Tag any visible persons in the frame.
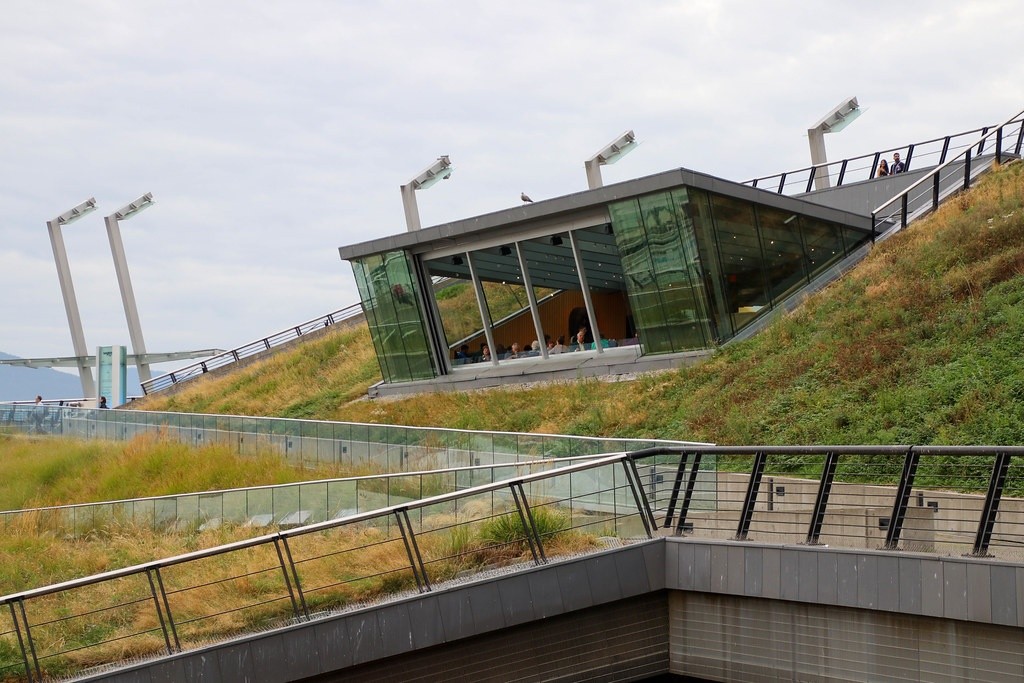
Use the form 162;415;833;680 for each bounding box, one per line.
877;159;889;177
97;396;109;409
889;153;906;175
33;396;46;434
454;328;621;363
